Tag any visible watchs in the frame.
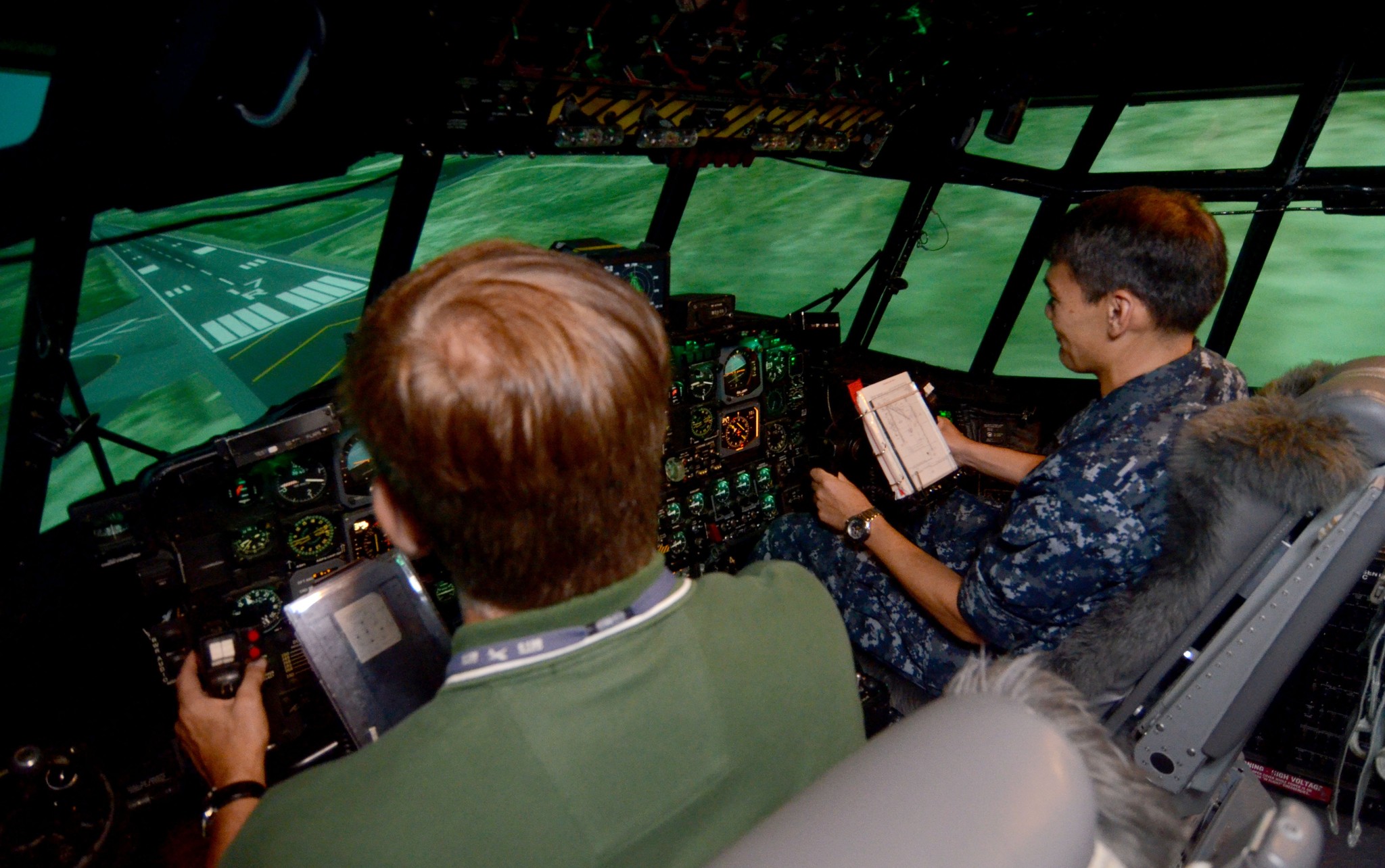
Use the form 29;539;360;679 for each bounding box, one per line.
200;780;266;838
843;508;881;550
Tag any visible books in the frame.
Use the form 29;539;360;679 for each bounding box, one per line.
856;370;958;500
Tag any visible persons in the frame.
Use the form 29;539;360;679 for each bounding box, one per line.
178;239;868;868
812;191;1250;697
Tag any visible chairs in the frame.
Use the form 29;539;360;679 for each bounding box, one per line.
1065;354;1384;820
706;649;1323;868
